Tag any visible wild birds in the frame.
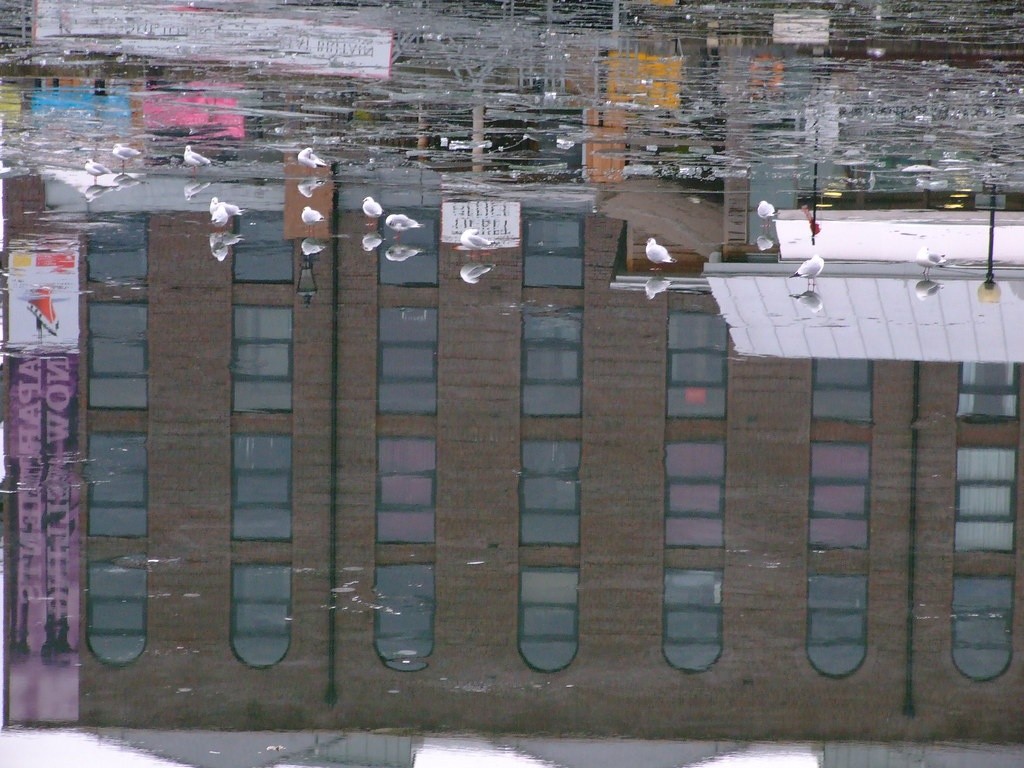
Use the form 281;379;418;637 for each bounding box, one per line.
645;238;677;269
84;170;148;202
301;206;329;232
300;233;329;257
207;197;245;226
915;246;945;276
183;145;211;170
756;228;773;251
83;143;143;181
362;225;426;263
788;285;825;317
209;231;246;262
644;276;672;300
459;255;497;284
460;227;495;249
786;253;825;285
757;199;779;227
183;177;211;200
914;276;944;302
297;146;328;173
297;173;329;198
362;196;425;239
